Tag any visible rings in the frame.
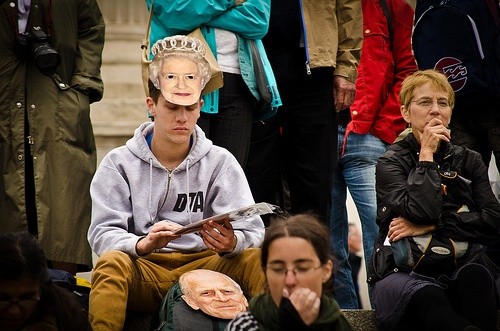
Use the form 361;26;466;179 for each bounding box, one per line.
211;247;216;251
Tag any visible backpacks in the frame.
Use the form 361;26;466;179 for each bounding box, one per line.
393;167;484;281
158;279;228;331
411;0;491;98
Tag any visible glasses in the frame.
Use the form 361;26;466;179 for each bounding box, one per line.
410;99;450;107
0;297;37;310
263;265;323;277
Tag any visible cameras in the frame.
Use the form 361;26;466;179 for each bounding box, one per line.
14;26;62;77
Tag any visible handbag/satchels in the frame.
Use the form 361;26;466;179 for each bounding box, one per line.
139;2;223;98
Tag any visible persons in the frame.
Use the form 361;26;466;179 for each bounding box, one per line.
146;1;499;308
367;71;500;331
0;0;105;267
0;231;94;331
87;36;264;331
225;214;355;331
